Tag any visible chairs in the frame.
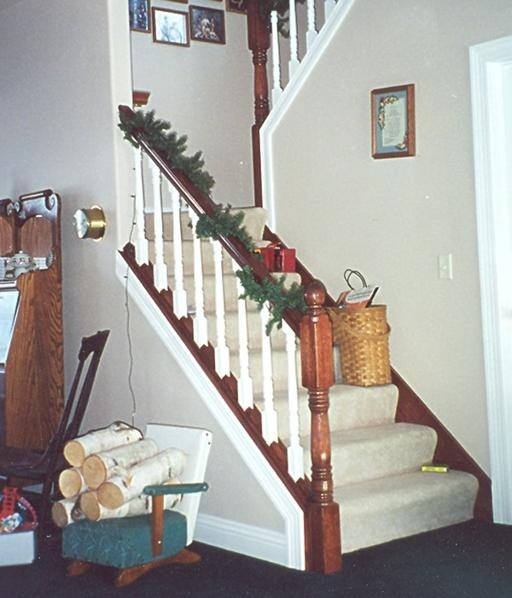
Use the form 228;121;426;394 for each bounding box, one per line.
1;331;107;560
62;425;215;586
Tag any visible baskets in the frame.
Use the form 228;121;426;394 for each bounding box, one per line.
327;306;392;386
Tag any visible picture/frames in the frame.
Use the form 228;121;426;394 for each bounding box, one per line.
129;0;248;48
371;83;416;159
